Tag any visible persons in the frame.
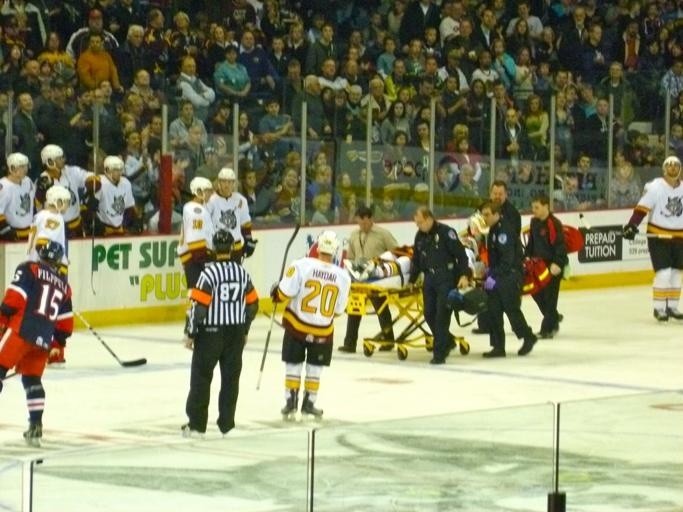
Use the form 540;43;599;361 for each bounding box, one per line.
27;186;71;368
305;180;569;364
0;240;74;438
181;229;258;439
176;168;258;299
270;232;351;420
622;156;683;322
1;2;682;242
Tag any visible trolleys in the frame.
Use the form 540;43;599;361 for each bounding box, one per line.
352;274;474;360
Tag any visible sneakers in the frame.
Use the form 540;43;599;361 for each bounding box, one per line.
343;258;379;283
180;422;205;433
22;420;43;440
653;307;683;321
336;344;356;354
377;342;394;352
429;313;566;364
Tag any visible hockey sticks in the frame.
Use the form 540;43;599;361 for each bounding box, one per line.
255;223;301;391
73;309;146;368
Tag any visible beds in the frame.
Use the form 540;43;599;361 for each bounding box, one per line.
347;246;546;360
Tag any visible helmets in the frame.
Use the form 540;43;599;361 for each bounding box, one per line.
661;155;681;170
39;144;64;166
5;152;28;167
44;184;71;205
316;231;339;255
38;241;63;261
216;166;236;180
189;176;212;194
103;155;124;171
212;231;233;248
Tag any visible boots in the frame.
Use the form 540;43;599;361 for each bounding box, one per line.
279;386;324;417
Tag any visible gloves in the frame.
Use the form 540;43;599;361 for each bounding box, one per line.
46;340;64;364
483;277;496;291
81;192;98;212
241;238;258;257
622;224;638;241
34;176;53;204
